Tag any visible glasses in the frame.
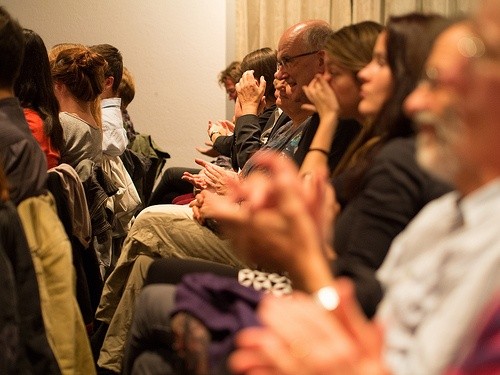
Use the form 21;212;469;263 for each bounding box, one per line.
278;51;318;70
